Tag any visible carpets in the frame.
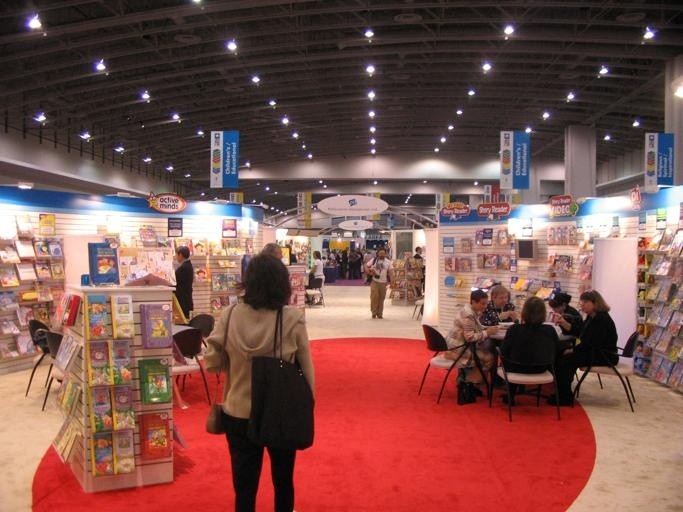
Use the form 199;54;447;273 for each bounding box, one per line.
32;337;595;512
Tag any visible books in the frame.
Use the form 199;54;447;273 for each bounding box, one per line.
54;294;82;462
632;201;683;395
85;295;171;476
87;243;120;285
104;224;170;279
0;213;64;359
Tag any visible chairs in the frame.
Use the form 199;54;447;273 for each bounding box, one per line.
418;324;492;403
571;330;639;413
307;278;325;309
487;337;565;422
42;331;62;411
412;296;424;320
171;292;221;410
25;319;53;397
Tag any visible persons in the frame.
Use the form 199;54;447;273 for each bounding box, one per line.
439;289;504;386
545;288;618;409
546;290;582;335
479;284;519;350
306;244;377;290
173;245;193;319
497;294;561;407
261;242;282;261
202;252;317;512
411;245;425;297
362;248;396;319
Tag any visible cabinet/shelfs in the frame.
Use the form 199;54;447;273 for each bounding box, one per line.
188;256;244;326
52;283;177;494
389;248;424;306
633;219;683;395
286;266;306;309
0;234;67;375
59;235;179;289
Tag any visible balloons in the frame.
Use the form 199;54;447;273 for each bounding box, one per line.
442;221;623;302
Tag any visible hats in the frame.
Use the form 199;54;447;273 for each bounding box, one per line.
549;292;571;308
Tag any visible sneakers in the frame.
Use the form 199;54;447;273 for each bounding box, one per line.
371;313;383;319
457;367;573;407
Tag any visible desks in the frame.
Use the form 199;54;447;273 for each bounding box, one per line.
306;288;321;295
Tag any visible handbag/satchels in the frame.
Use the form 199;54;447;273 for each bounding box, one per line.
248;304;315;453
206;402;229;433
366;271;372;282
